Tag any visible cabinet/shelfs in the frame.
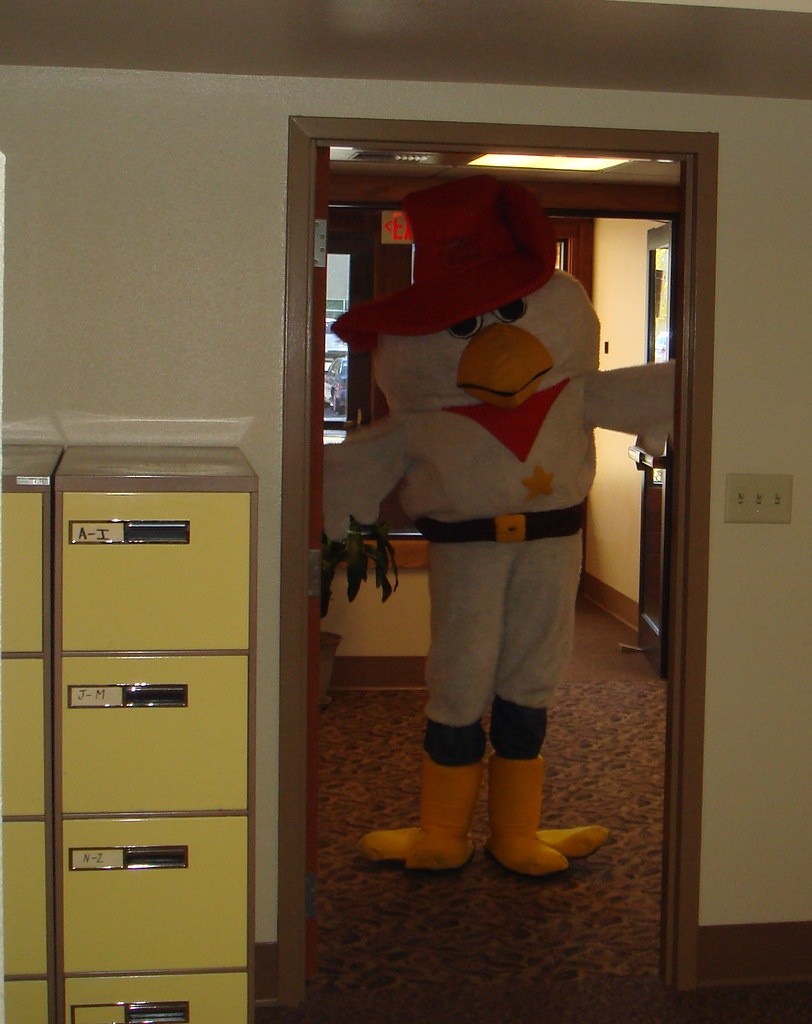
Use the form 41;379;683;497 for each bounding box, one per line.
0;443;65;1024
53;445;260;1024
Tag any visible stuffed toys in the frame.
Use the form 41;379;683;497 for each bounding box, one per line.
323;174;676;876
